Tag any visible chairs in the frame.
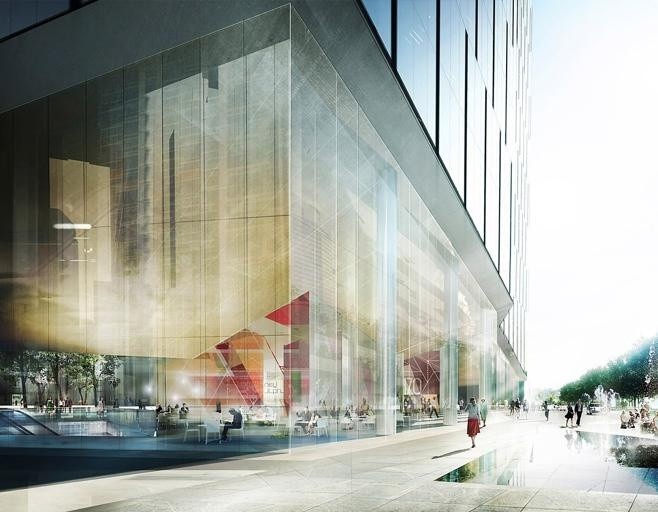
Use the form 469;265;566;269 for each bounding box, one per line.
156;406;376;445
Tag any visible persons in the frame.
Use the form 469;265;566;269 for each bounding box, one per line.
563;402;573;427
573;398;583;426
617;400;657;435
513;398;521;420
508;400;514;416
458;398;464;414
479;398;488;428
541;400;549;421
521;399;529;420
459;396;480;448
426;398;431;413
428;396;439;419
403;398;408;416
406;396;413;416
